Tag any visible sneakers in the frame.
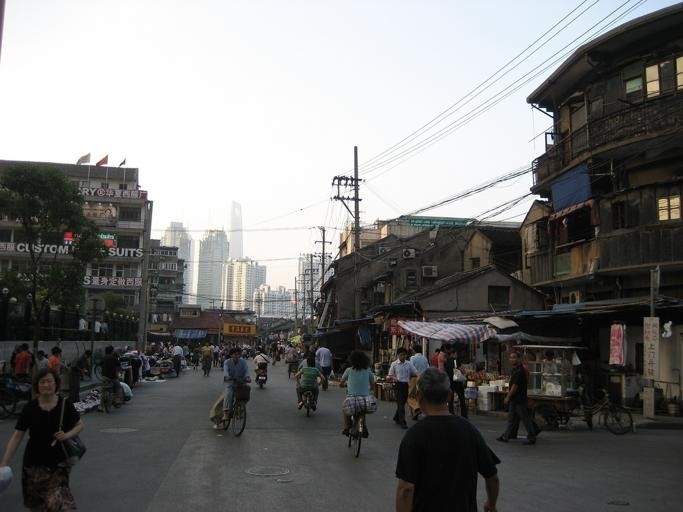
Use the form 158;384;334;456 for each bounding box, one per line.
221;416;229;421
298;402;304;410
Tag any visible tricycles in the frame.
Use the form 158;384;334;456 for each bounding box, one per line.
0;358;32;420
489;375;634;436
288;359;301;380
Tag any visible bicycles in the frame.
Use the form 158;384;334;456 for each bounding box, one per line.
217;374;254;438
92;352;104;381
339;380;373;459
295;375;320;418
99;366;127;414
172;342;264;377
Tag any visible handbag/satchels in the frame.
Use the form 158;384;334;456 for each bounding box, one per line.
453;368;466;382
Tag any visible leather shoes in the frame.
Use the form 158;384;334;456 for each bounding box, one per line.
496;436;507;442
342;430;349;436
401;422;408;429
393;417;399;424
523;437;537;445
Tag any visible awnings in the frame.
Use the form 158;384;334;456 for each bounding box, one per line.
175;328;207;338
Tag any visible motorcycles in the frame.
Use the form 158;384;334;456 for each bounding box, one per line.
252;361;268;388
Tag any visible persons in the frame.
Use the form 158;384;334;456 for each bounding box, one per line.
241;339;286;384
394;366;501;512
76;346;126;412
1;367;84;512
223;348;251;421
495;350;559;445
284;343;333;412
340;350;375;438
145;341;240;377
387;343;468;429
9;341;64;378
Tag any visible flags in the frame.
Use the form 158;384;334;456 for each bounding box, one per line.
76;152;90;166
118;159;124;167
95;155;107;166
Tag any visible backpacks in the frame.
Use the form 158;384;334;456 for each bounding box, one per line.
50;397;86;464
